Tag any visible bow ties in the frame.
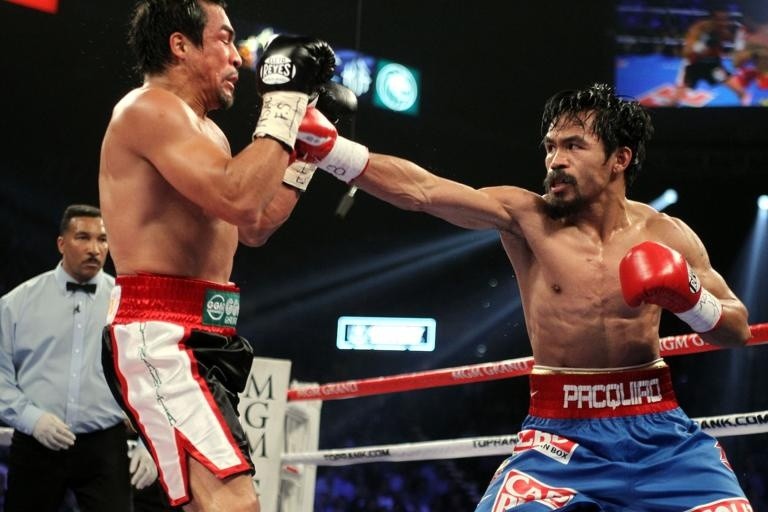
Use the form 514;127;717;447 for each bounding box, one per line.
66;282;96;293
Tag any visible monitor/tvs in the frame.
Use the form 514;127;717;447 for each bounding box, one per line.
605;0;768;113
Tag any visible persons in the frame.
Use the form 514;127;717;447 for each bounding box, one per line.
0;205;157;512
726;5;768;105
671;7;752;107
302;82;753;510
100;0;359;512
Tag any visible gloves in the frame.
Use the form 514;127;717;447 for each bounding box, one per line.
287;107;369;183
282;81;357;193
129;438;158;489
619;242;724;334
252;30;336;150
33;414;76;450
